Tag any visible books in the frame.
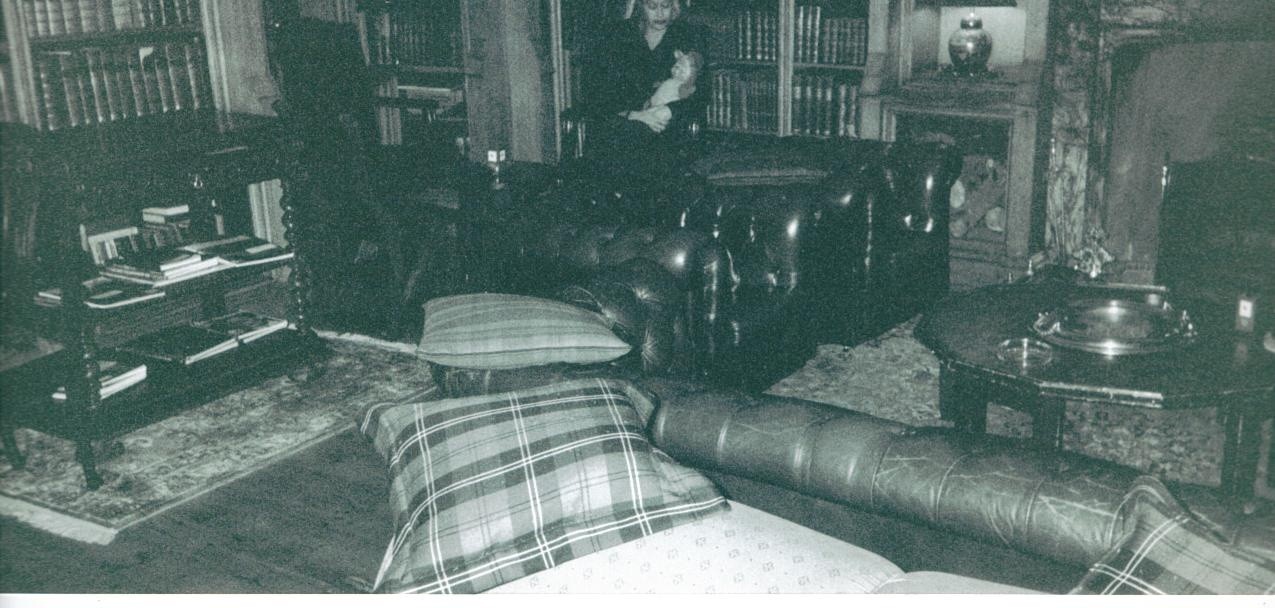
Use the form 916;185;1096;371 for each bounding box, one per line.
0;0;462;403
696;4;867;138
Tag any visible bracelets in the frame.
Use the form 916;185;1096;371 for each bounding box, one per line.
627;108;633;119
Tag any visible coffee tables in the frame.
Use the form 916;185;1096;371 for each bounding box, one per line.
912;274;1275;496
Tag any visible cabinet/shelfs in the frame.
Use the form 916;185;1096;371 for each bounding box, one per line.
557;0;872;141
0;0;223;132
25;107;323;440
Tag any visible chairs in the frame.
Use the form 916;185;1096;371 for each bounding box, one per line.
0;117;99;494
1154;152;1275;298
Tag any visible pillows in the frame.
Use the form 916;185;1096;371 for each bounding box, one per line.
361;371;735;594
1066;470;1275;595
410;279;638;368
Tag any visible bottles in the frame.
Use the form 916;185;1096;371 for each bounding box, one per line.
948;15;993;76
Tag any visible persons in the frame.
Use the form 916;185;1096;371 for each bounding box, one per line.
589;1;708;170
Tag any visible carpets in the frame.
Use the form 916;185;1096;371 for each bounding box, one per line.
0;311;433;548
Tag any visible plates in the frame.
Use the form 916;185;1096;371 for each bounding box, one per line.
1033;299;1198;356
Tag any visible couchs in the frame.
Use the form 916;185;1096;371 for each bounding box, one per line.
314;115;1275;595
